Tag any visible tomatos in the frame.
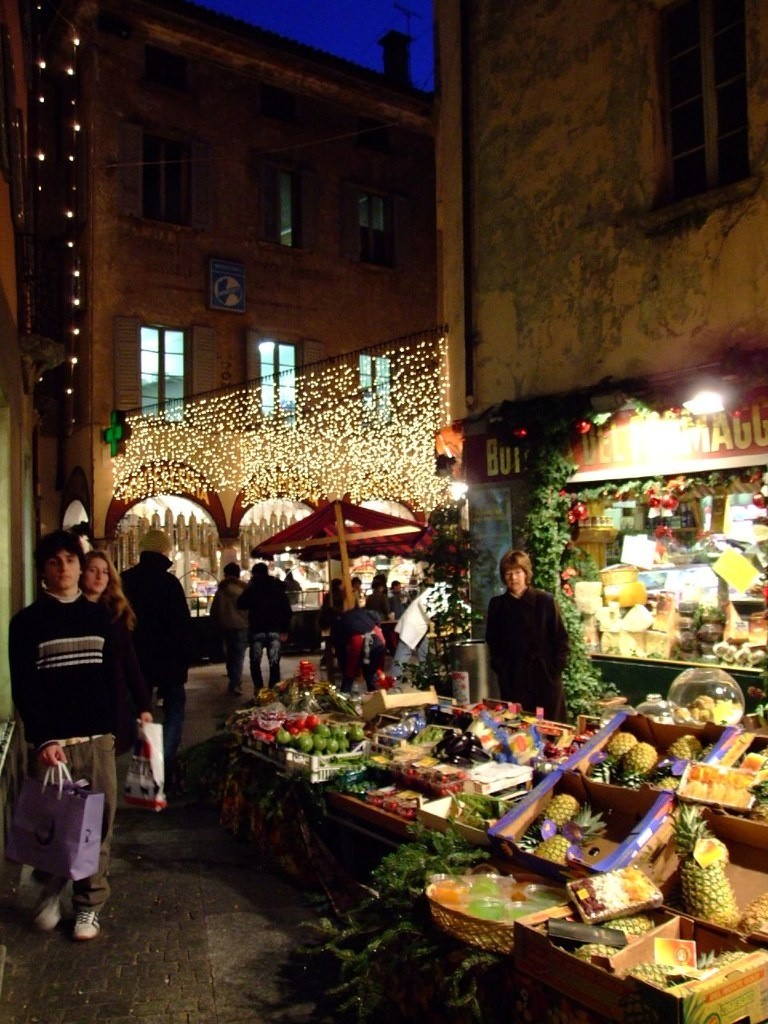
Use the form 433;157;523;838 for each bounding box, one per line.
366;766;468;819
250;714;320;743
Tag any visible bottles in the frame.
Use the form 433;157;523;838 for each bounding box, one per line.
668;668;744;726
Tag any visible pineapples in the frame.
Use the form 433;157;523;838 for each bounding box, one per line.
520;730;768;993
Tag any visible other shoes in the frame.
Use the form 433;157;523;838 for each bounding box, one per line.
230;686;244;696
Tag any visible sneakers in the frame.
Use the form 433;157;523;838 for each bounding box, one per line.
73;910;102;941
32;896;62;930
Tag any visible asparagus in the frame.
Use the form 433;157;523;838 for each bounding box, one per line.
284;683;358;717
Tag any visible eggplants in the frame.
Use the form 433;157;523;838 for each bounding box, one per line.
431;730;492;767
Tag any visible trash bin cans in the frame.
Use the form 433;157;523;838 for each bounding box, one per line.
455;639;490;704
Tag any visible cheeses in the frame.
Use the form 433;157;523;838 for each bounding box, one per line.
571;565;676;661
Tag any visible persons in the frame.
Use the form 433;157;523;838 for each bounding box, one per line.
284;568;302;604
237;563;292;697
120;529;193;801
78;550;154;786
210;563;249;695
485;550;571;723
318;574;417;693
9;530;119;941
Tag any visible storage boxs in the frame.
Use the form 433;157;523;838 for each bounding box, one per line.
362;684;768;1024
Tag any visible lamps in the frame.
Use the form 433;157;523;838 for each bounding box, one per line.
682;380;725;415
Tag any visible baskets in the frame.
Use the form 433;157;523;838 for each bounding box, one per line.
424;875;569;955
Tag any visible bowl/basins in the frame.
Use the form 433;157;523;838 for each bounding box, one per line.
428;871;570;923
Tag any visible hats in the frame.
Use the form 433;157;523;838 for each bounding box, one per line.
141;530;171;554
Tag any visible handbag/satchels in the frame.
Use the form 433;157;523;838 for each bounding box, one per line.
123;719;169;813
5;759;106;882
